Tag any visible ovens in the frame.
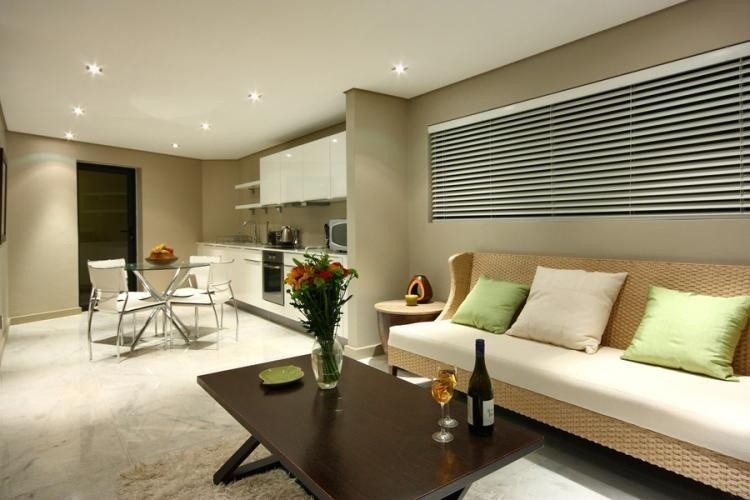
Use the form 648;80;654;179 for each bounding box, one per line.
262;250;284;307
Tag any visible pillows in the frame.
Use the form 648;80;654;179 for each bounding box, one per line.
450;275;530;334
505;265;628;353
621;284;749;382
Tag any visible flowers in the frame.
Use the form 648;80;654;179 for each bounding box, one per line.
284;249;360;384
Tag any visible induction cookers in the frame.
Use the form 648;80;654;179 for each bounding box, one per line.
264;246;305;249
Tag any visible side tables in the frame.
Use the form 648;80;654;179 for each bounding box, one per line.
374;298;446;355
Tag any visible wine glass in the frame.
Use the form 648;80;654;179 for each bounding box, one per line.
431;378;454;443
438;368;458;428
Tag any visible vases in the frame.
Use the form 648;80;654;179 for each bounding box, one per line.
311;336;343;390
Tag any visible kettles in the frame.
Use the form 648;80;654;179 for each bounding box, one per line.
280;225;294;246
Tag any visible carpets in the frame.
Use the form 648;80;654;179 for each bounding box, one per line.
113;430;506;499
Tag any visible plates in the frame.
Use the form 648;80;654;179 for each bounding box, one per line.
259;365;304;385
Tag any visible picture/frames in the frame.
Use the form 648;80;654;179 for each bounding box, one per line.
1;148;6;244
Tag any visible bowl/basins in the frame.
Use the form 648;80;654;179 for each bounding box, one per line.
144;257;178;266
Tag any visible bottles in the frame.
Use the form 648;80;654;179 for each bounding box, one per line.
466;338;494;435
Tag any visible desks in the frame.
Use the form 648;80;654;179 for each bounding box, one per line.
124;261;210;352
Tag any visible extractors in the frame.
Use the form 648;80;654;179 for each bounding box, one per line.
263;203;329;208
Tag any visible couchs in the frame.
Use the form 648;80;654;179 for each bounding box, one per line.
387;250;750;500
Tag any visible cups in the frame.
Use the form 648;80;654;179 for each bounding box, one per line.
405;294;419;306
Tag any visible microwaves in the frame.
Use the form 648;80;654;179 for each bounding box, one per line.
329;219;347;253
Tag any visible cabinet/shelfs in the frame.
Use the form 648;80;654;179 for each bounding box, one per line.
196;245;216;284
303;131;347;203
234;180;260;210
216;246;262;304
283;252;348;349
260;144;303;208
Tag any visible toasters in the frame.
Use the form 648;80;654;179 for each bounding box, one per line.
269;231;282;245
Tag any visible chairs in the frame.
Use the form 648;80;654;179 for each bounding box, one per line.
163;256;221;338
87;258;166;345
87;264;166;359
168;260;238;349
